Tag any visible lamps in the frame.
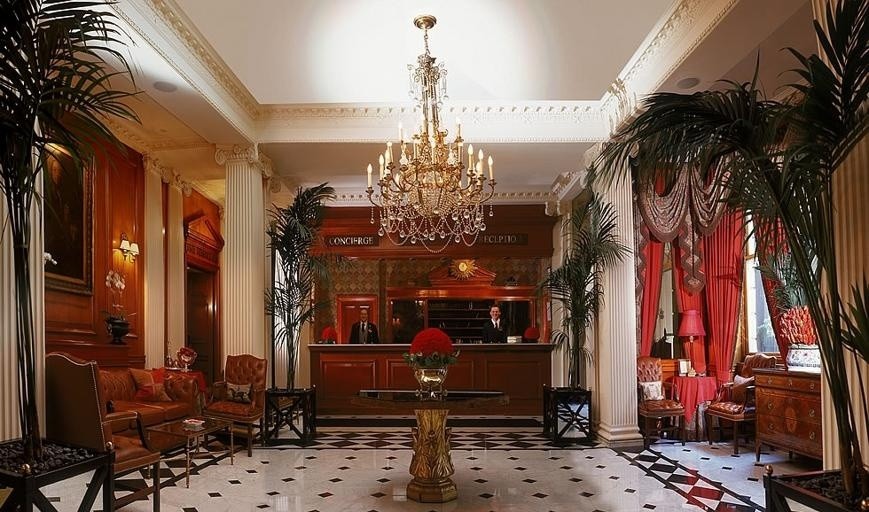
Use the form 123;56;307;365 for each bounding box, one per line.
363;12;498;254
119;232;139;263
677;309;707;378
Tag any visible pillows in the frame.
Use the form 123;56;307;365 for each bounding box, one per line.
128;368;172;403
224;380;254;405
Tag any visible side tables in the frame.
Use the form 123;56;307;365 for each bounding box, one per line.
671;374;718;446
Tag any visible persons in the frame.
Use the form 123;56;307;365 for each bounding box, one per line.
43;154;84;273
482;304;508;343
349;308;380;344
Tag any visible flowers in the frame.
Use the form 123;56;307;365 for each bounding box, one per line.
321;326;337;343
403;326;462;367
524;326;540;340
778;305;817;344
102;267;137;321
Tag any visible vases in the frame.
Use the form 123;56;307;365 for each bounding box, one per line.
104;317;129;345
413;368;449;398
784;344;821;374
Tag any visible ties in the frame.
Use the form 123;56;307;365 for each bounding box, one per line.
495;323;497;328
361;324;365;331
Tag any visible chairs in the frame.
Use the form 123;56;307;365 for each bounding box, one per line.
42;351;162;512
705;355;778;454
202;353;268;458
638;356;688;448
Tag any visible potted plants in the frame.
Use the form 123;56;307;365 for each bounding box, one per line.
528;181;634;444
262;180;336;448
0;0;146;512
583;2;869;512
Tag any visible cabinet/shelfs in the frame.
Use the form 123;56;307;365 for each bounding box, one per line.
754;368;822;466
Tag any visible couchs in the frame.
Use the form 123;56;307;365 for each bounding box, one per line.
96;363;208;440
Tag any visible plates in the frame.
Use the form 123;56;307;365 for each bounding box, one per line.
183;427;205;432
183;418;204;427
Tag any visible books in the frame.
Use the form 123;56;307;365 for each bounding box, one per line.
507;334;524;343
